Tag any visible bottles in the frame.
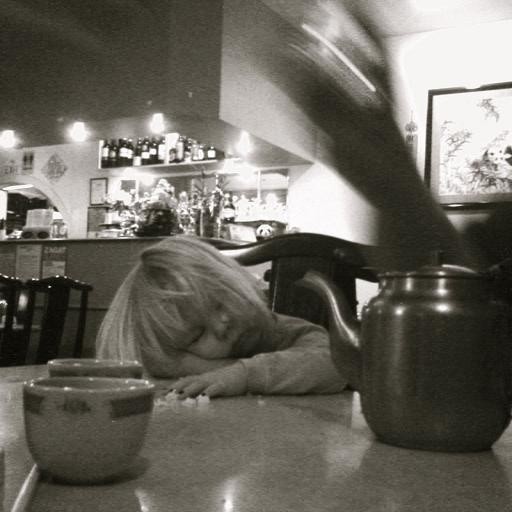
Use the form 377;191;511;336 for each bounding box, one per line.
222;194;234;221
199;207;212;237
100;135;218;169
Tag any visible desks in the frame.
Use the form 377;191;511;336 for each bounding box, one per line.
1;363;511;511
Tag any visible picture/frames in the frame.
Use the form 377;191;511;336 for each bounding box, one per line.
424;81;512;211
90;178;108;206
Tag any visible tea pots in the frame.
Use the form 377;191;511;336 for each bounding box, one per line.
294;252;512;452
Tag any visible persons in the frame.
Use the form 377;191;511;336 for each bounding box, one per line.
95;234;349;401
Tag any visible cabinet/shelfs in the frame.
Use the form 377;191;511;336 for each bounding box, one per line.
98;140;290;241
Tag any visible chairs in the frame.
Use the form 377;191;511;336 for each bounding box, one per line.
24;275;98;363
219;233;445;332
0;274;28;367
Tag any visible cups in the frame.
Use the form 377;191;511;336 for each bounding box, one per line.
21;376;156;485
47;358;144;379
